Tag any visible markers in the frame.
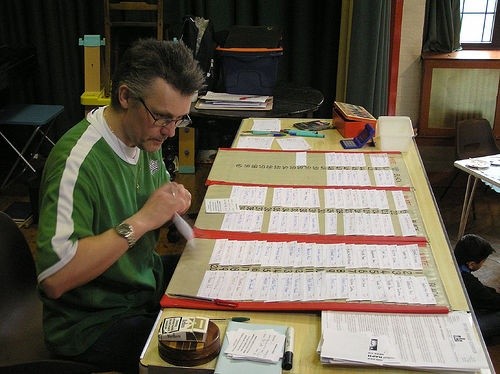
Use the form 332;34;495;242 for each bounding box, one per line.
282;326;294;370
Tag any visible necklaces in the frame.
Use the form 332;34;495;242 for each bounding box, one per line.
104;108;140;188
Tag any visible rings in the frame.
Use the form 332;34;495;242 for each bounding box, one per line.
171;192;175;196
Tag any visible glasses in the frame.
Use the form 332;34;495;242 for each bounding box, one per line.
138;97;192;127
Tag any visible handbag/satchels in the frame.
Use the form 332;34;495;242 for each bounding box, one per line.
177;15;216;95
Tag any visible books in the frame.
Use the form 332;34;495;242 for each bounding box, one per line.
199;91;269;107
214;321;288;374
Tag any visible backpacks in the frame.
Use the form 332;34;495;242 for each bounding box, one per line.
215;25;287;96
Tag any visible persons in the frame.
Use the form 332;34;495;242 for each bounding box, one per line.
454;234;500;337
34;40;209;374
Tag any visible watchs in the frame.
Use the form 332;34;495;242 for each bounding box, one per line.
115;223;136;248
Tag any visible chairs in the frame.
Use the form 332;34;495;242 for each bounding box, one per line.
438;119;500;221
0;211;107;374
217;24;284;91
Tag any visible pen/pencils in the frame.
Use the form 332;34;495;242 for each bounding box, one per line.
209;316;250;322
253;129;325;138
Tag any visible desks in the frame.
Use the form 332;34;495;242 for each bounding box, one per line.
138;115;496;374
454;154;500;241
189;87;325;150
0;103;65;191
